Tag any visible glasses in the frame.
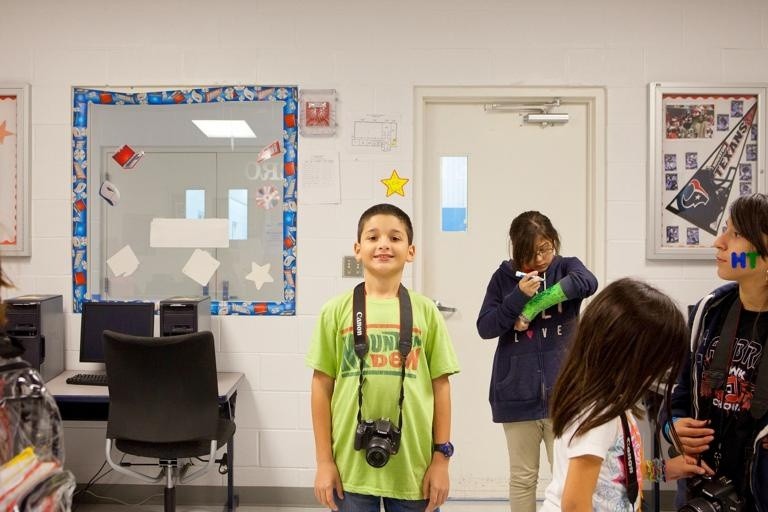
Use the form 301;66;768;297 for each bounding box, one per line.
532;239;554;256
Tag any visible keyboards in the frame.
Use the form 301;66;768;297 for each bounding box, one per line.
66;374;109;385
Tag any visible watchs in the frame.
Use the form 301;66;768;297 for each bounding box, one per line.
431;439;456;459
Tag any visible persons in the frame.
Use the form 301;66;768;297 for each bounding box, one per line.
662;100;758;247
303;203;461;512
535;276;717;510
475;209;600;511
656;190;767;510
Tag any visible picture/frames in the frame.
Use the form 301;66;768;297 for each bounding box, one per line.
0;81;34;260
644;79;768;263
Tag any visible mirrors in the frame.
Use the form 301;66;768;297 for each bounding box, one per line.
71;83;300;317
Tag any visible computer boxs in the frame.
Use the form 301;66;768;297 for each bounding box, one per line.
3;294;64;385
160;296;210;337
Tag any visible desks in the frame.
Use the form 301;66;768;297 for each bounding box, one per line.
45;370;243;510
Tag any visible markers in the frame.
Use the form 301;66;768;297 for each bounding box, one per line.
515;271;546;282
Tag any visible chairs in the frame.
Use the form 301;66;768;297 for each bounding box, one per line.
93;328;236;511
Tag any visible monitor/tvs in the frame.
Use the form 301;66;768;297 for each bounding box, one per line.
79;302;154;363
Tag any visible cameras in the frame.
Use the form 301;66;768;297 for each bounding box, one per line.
677;472;743;512
353;419;401;468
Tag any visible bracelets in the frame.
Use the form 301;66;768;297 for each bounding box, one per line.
663;416;681;443
644;457;668;483
